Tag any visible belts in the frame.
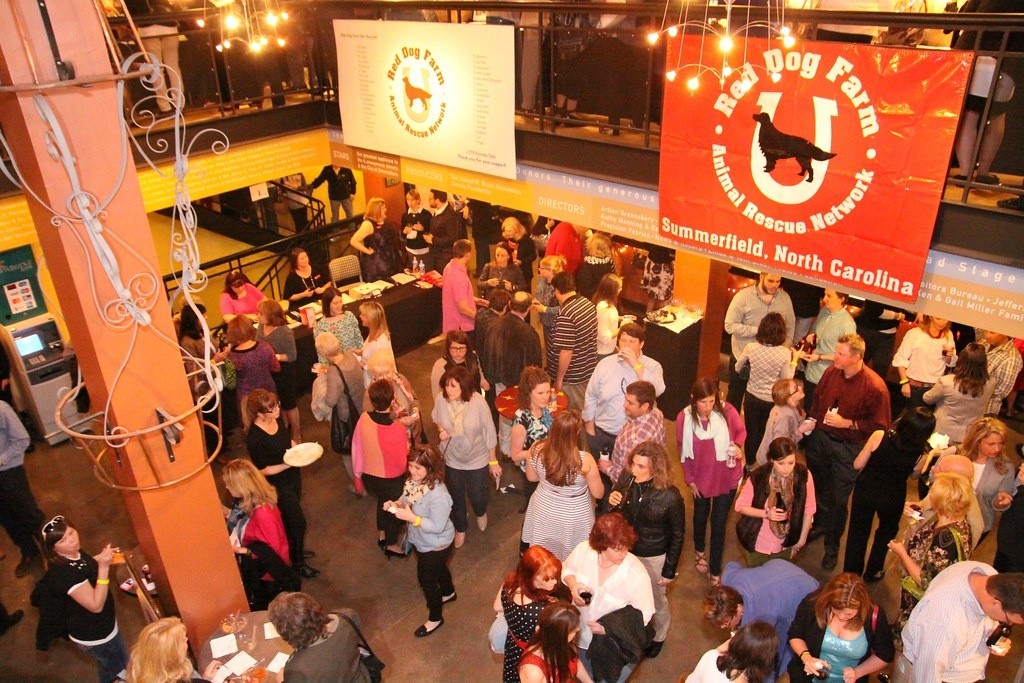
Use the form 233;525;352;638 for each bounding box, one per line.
908;377;935;388
823;430;852;444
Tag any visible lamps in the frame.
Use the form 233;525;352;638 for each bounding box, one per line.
646;0;795;94
195;0;289;53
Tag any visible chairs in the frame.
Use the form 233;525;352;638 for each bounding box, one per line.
329;254;366;291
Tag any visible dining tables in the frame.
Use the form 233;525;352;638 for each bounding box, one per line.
643;306;701;421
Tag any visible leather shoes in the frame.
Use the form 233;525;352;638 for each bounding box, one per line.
15;555;35;578
218;442;243;465
302;552;320;579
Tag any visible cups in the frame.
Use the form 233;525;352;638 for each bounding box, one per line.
803;420;812;436
814;661;831;680
580;592;592;605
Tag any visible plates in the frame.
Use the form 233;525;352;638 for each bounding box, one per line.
283;442;324;467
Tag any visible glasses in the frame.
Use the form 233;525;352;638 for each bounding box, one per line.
449;346;467;352
790;382;798;396
265;400;281;413
42;515;64;541
995;597;1015;628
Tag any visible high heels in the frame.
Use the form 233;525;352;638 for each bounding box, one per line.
377;539;411;561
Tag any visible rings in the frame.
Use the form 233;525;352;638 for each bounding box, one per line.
442;436;445;439
498;469;501;472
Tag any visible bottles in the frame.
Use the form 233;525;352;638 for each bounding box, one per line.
806;334;817;354
823;398;840;425
793;339;804;350
419;260;425;275
412;257;418;273
409;397;418;416
727;441;736;468
607;476;635;513
219;333;226;353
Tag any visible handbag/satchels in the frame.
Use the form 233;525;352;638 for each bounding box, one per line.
899;526;964;601
330;363;360;455
328;612;385;683
740;360;751;382
224;359;236;389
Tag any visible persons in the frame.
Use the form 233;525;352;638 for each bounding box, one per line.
675;271;1024;683
116;617;222;683
950;0;1024;188
308;165;357;223
62;339;91;420
181;190;686;683
552;0;639;127
0;400;45;578
281;173;311;232
0;602;24;635
30;515;128;683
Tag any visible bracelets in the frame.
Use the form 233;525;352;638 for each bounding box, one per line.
899;380;909;384
849;420;855;430
800;650;809;658
766;508;769;517
439;427;445;432
97;579;110;585
321;288;326;291
634;365;642;369
489;462;498;464
790;362;797;365
413;517;420;526
818;354;821;362
275;353;281;361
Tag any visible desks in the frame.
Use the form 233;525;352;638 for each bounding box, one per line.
218;269;442;398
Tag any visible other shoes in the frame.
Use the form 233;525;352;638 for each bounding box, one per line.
476;513;488;531
414;618;444;637
442;592;457;605
863;569;885;582
0;609;24;636
347;484;367;498
806;525;826;545
645;640;664;658
822;553;838;571
454;530;465;548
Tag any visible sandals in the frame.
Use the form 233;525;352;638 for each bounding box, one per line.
710;574;720;587
694;550;708;574
